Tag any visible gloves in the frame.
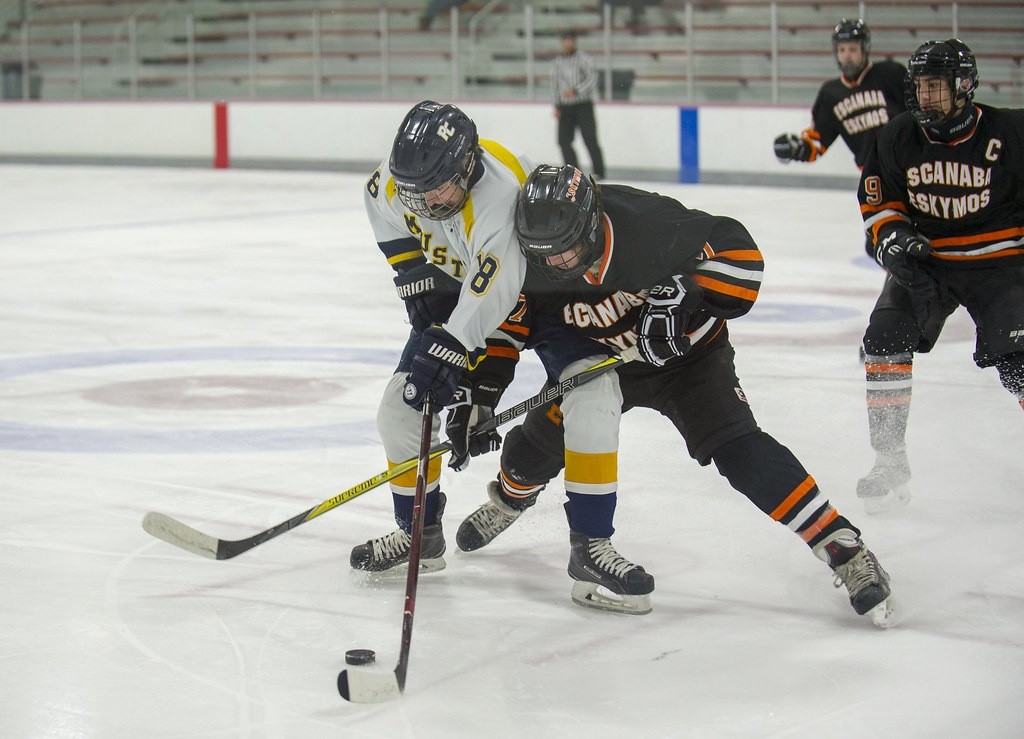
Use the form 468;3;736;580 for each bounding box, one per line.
394;265;458;336
775;134;814;162
445;379;505;471
872;231;935;298
636;269;705;367
403;324;472;415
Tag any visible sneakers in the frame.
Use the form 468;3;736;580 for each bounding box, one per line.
350;491;446;582
811;536;894;629
563;501;656;616
856;453;910;516
454;472;527;555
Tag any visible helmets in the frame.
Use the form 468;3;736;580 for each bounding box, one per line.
901;37;978;130
390;99;480;222
832;17;873;81
515;164;599;283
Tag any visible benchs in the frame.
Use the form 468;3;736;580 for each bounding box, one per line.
0;0;1024;108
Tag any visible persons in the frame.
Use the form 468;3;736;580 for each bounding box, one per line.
550;30;607;179
351;97;656;615
774;17;917;257
855;40;1024;499
456;163;894;629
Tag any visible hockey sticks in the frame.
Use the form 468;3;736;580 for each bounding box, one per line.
334;390;436;703
140;343;644;563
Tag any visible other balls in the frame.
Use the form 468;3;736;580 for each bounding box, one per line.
345;649;376;666
